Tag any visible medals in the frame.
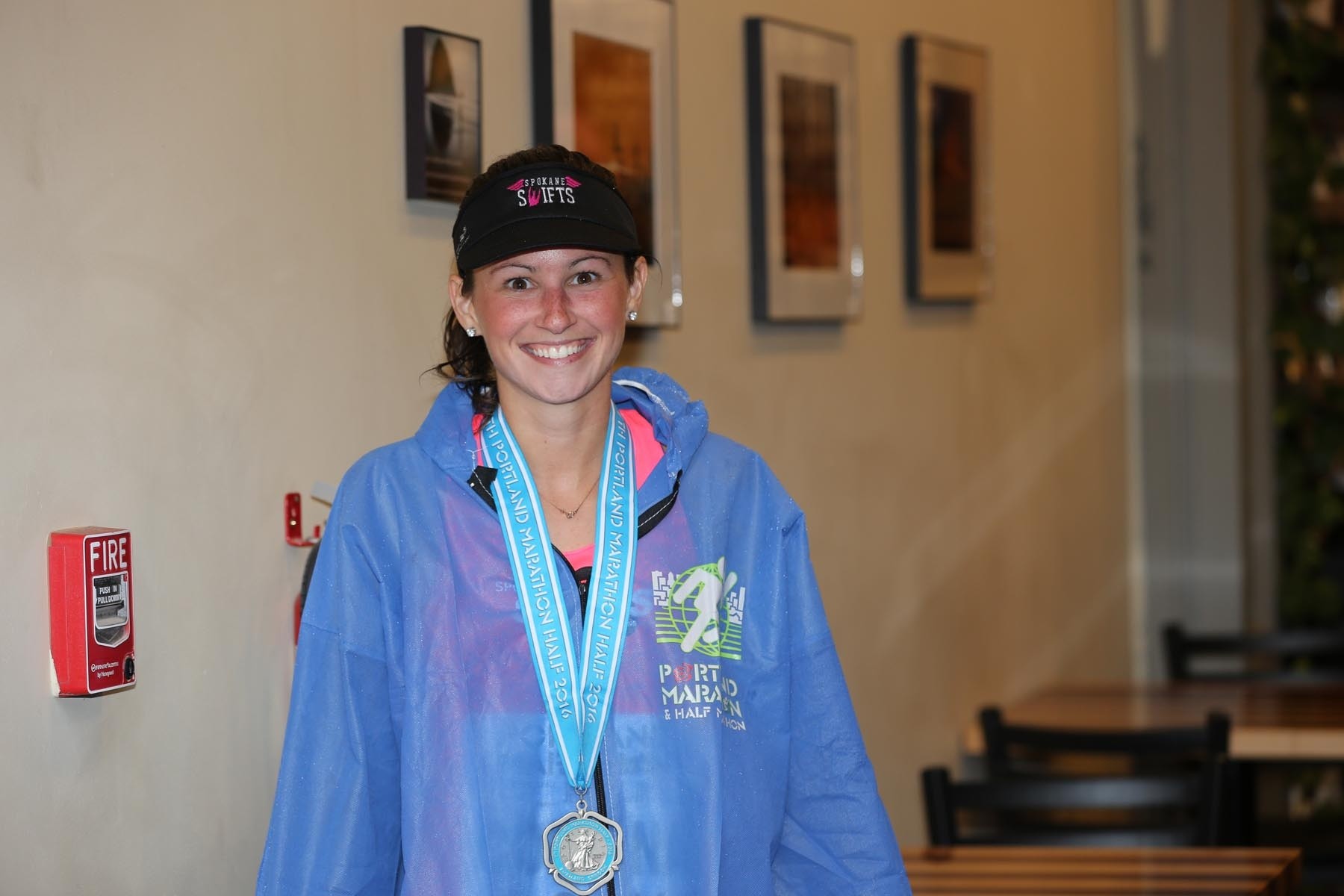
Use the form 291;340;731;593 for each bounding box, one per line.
543;801;623;896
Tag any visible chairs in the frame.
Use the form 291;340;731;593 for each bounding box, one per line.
913;587;1344;857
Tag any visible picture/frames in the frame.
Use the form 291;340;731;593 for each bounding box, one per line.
735;11;875;334
400;24;486;215
893;29;996;311
531;0;685;342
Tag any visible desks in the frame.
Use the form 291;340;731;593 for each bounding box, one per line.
943;668;1344;764
897;830;1307;896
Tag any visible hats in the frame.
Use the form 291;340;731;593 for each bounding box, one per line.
452;162;637;277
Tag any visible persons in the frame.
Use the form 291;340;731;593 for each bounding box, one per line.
255;147;913;896
